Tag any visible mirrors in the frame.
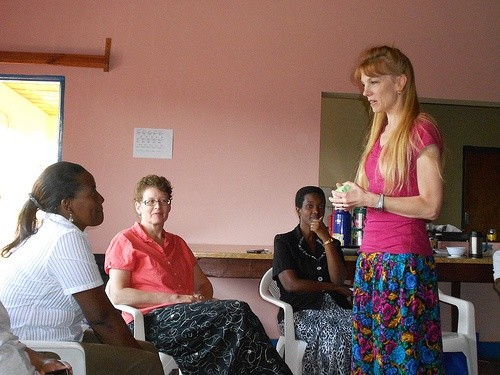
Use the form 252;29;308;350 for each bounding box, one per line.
314;86;500;243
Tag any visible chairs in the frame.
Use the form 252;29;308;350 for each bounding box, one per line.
260;259;481;375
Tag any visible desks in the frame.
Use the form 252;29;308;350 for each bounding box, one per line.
193;249;500;347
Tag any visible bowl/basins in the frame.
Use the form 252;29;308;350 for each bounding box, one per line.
446;246;466;256
433;249;448;254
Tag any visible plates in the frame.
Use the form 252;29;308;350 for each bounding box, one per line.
448;256;464;258
434;254;448;257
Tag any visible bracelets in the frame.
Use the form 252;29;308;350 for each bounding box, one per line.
321;238;334;246
375;193;384;211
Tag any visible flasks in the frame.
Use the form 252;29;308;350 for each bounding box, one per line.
469;231;482;258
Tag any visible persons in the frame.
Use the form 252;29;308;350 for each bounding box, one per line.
1;302;73;375
104;174;298;375
328;45;446;375
0;161;165;375
273;186;354;375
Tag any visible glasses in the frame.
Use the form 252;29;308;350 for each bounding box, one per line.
140;198;173;206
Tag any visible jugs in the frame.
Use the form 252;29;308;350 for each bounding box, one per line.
487;228;496;243
349;205;365;246
328;209;336;238
334;207;350;245
